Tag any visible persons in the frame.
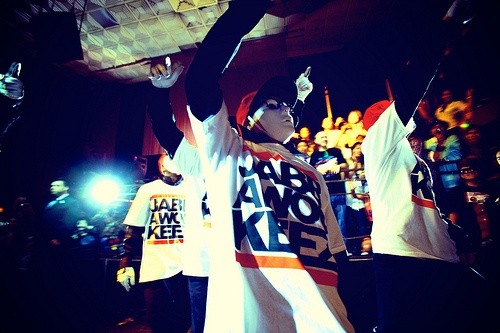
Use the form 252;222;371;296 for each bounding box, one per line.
118;149;182;332
184;0;357;333
360;0;474;333
0;60;25;135
147;56;313;332
289;89;498;257
39;180;75;331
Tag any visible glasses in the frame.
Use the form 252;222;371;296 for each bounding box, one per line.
251;97;293;114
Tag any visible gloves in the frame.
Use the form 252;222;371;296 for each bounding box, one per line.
148;56;184;88
295;66;313;103
117;267;135;292
0;63;24;99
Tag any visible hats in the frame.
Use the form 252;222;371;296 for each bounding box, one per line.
362;100;392;131
236;76;298;127
